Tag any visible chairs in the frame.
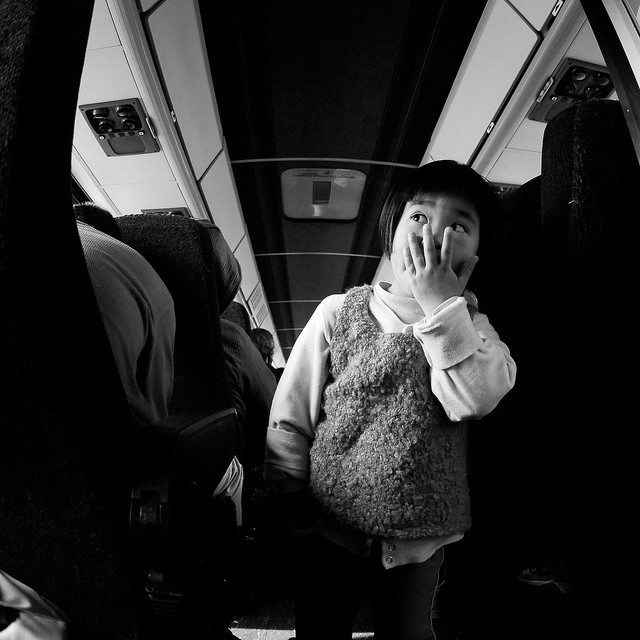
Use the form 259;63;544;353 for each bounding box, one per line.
542;101;639;324
0;2;241;639
116;217;236;410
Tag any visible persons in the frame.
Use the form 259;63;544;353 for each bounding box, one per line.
215;319;277;468
261;160;519;638
248;329;285;385
70;202;244;639
